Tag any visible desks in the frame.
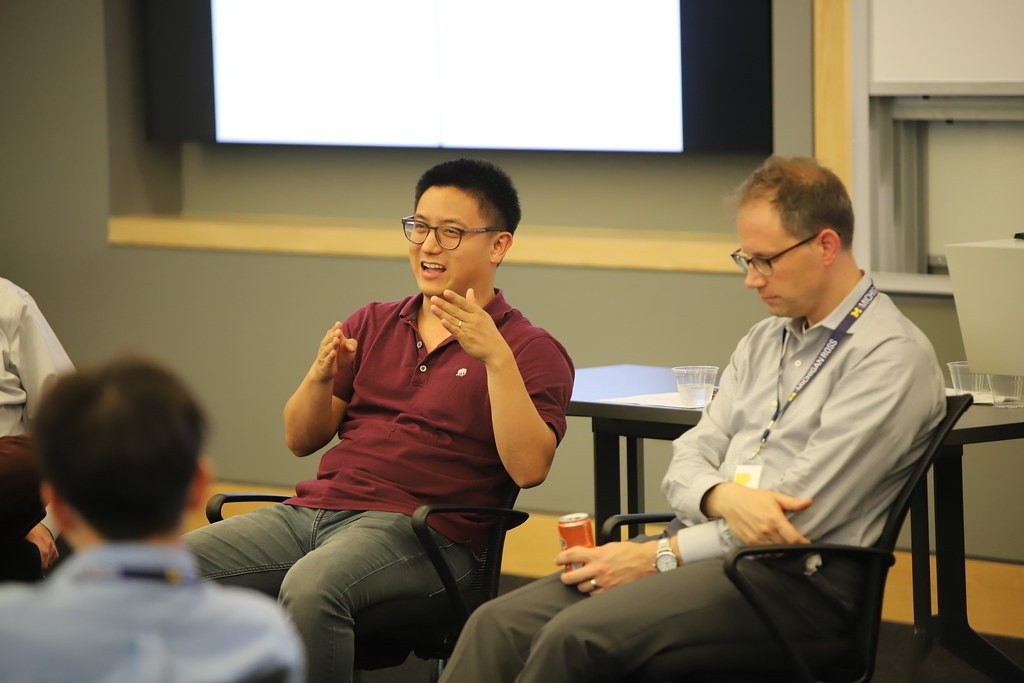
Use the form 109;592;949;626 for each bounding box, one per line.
565;363;1024;682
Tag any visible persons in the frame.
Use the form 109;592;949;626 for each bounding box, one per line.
1;360;306;683
178;158;574;683
437;154;946;683
1;277;81;571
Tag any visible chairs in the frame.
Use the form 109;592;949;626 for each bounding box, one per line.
204;493;532;683
602;392;974;683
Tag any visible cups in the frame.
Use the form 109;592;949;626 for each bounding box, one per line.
672;366;719;410
987;374;1024;408
947;360;983;396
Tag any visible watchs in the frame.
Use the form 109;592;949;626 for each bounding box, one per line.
652;537;678;573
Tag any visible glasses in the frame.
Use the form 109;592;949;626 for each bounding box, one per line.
400;216;504;250
731;232;841;277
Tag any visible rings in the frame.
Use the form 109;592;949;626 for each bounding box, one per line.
459;321;462;328
590;579;598;589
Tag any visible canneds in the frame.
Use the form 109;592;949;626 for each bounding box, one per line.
558;512;596;577
